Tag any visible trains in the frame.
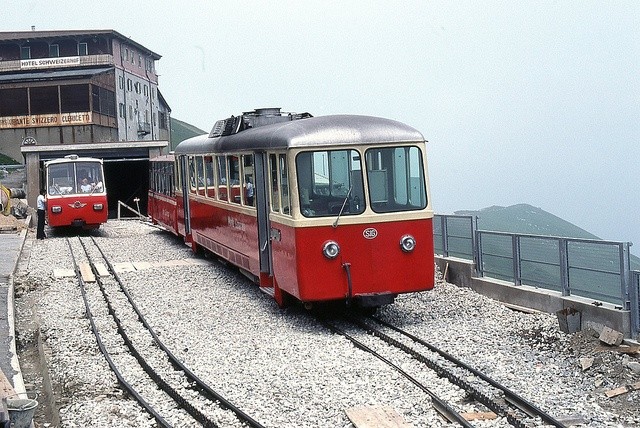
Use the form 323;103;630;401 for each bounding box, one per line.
147;106;435;310
44;154;108;229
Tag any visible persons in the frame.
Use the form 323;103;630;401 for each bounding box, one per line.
245;176;253;206
36;189;47;239
